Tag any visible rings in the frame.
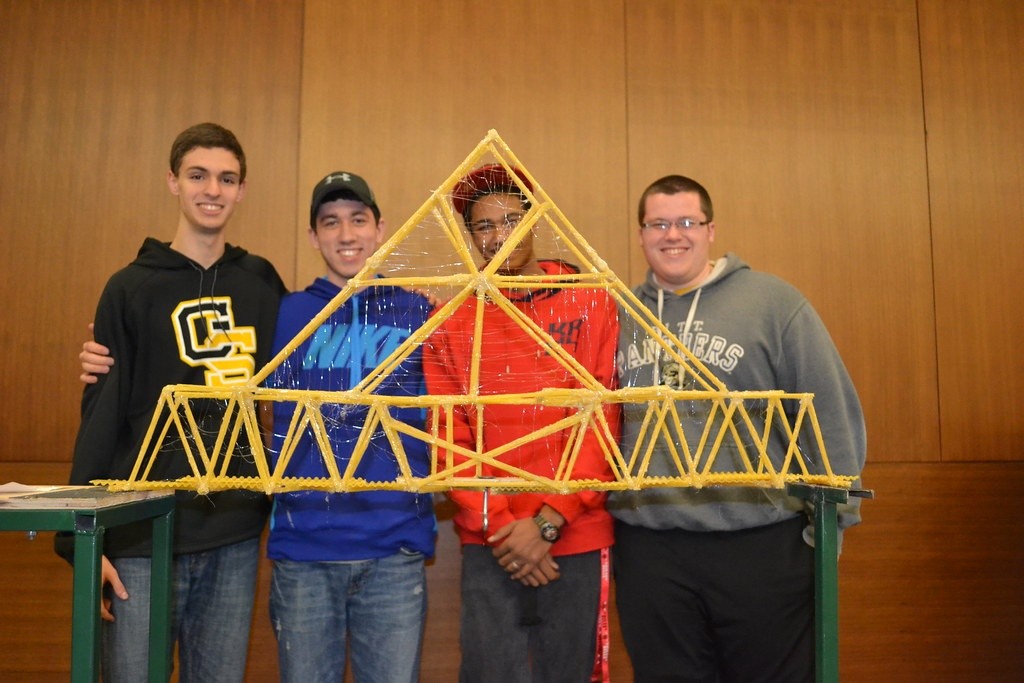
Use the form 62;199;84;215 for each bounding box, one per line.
512;561;521;571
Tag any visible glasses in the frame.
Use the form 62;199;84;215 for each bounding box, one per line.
642;219;709;232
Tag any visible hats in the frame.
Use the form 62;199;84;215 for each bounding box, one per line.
311;171;376;214
452;162;533;215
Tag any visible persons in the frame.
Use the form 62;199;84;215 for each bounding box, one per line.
78;170;441;683
604;174;867;683
423;161;623;683
53;121;439;683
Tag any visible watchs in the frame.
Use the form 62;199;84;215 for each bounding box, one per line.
532;513;561;542
